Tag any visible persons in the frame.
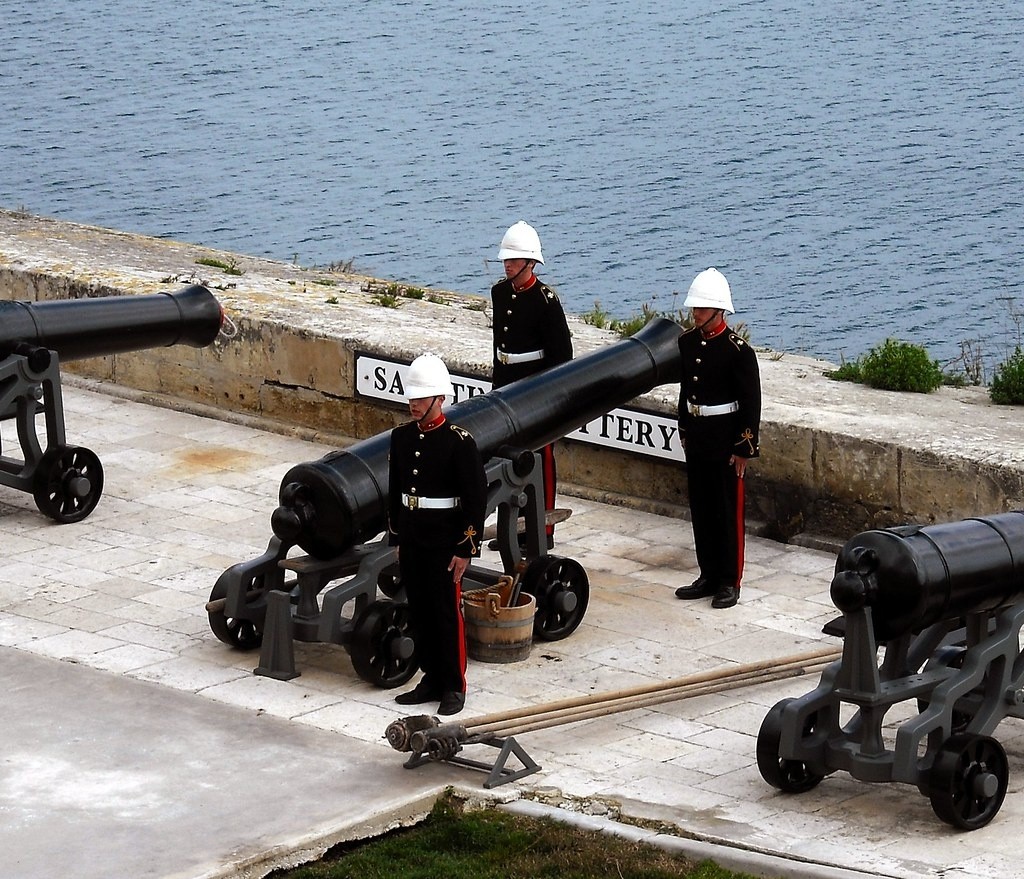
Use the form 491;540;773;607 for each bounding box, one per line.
388;353;487;716
675;267;762;609
488;220;572;558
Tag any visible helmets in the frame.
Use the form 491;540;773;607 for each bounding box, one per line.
684;267;735;314
404;352;456;400
498;221;545;266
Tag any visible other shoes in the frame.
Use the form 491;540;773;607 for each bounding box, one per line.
488;540;500;551
519;535;554;551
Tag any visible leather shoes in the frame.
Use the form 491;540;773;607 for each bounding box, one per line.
395;685;442;705
675;578;717;600
437;691;465;715
711;585;740;609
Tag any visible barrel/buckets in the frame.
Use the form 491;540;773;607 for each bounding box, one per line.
462;575;536;664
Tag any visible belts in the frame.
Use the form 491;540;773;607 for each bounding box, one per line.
687;398;739;417
496;346;544;365
401;493;461;511
